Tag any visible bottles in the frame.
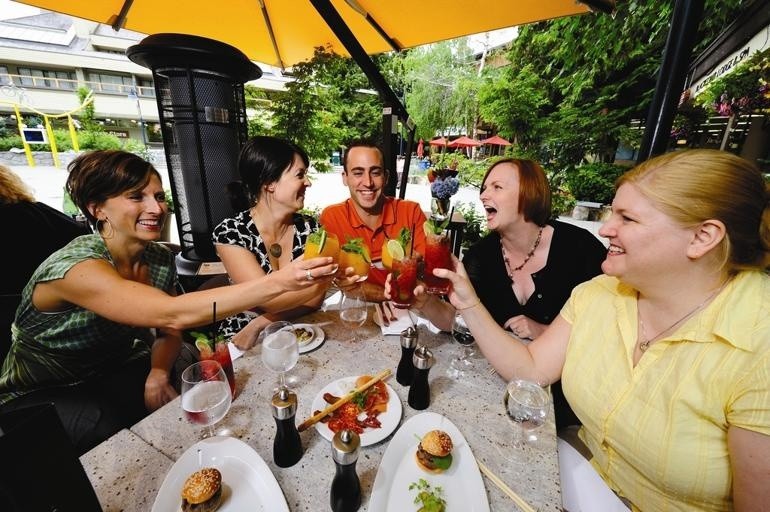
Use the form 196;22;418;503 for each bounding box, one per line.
396;326;419;386
330;428;362;511
408;345;434;410
270;388;304;467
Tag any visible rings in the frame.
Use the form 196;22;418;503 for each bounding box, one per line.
306;269;315;281
515;328;520;334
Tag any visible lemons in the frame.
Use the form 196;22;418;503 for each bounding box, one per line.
196;337;210;351
423;220;435;237
387;239;406;260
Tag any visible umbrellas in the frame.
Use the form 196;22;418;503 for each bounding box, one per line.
428;137;451;155
481;135;512;156
417;138;425;158
15;0;597;204
448;136;481;158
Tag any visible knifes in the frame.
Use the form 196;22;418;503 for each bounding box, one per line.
378;300;390;327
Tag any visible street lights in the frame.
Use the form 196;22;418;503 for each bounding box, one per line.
398;93;406;156
128;88;149;163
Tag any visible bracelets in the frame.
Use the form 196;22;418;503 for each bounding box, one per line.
452;300;483;310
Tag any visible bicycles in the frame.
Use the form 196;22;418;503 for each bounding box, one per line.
1;76;34;107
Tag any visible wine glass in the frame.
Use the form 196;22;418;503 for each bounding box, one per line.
338;286;368;351
180;360;239;438
261;320;299;392
495;369;551;463
447;309;475;372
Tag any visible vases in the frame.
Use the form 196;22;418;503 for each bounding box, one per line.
437;199;451;216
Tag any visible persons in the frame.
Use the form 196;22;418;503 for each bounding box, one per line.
437;148;770;511
0;146;337;464
446;157;613;430
210;134;328;358
318;141;438;307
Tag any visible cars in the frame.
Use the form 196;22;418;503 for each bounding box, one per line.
147;124;163;142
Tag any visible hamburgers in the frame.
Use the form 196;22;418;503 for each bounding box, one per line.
415;430;453;474
181;467;222;512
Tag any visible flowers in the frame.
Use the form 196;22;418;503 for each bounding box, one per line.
422;152;464;204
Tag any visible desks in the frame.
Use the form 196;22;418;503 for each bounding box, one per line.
424;212;468;260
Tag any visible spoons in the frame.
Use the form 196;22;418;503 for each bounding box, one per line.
384;301;398;322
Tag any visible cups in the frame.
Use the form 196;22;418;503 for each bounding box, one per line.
381;235;413;270
339;245;371;284
424;234;453;294
304;232;338;269
390;248;417;309
200;343;236;397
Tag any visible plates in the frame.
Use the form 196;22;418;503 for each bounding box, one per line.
277;323;323;353
310;376;403;447
151;436;290;512
286;324;314;347
365;411;491;511
372;309;418;328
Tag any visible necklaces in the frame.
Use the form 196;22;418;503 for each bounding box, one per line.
252;220;294;258
635;274;742;351
496;226;544;280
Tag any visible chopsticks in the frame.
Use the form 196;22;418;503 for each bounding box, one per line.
474;456;537;511
